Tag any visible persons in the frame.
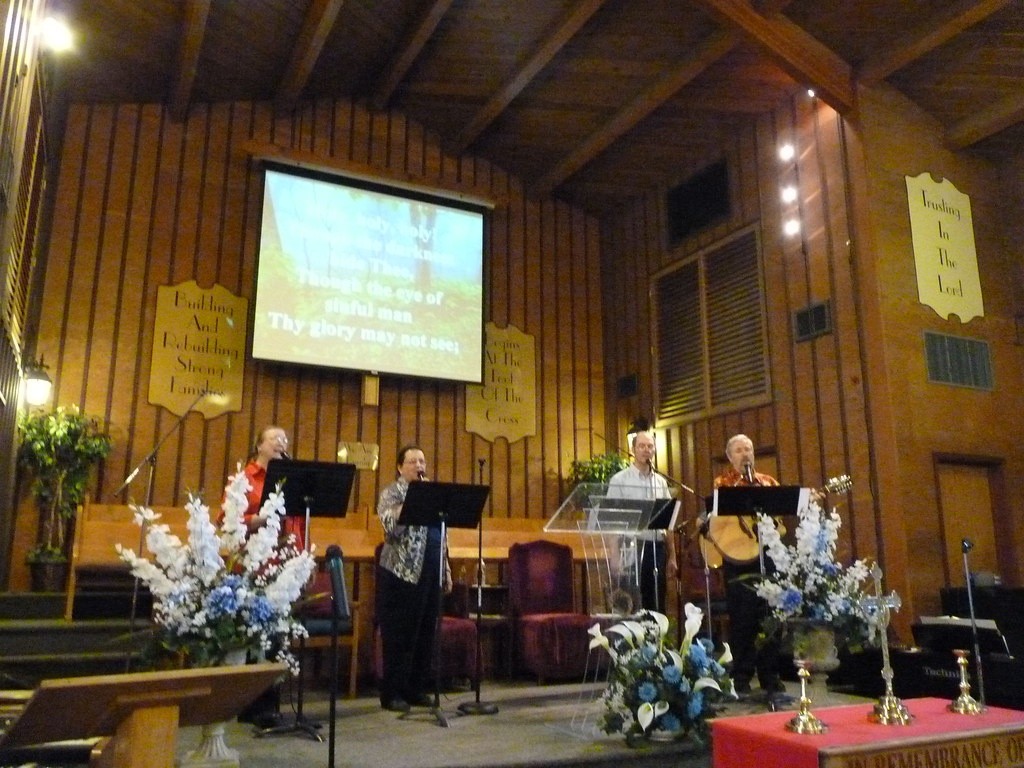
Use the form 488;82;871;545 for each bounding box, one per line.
604;430;678;612
216;424;318;732
696;433;827;710
375;444;453;713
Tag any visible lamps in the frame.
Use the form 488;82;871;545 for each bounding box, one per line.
21;353;52;405
626;414;652;456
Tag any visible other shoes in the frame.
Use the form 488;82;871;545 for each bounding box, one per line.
734;679;752;697
760;678;786;692
239;711;284;728
406;693;435;706
382;697;409;711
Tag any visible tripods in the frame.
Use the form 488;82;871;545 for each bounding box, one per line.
257;459;356;744
396;480;490;729
716;483;800;713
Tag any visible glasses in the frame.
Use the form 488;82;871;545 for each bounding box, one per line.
261;437;288;444
401;459;426;465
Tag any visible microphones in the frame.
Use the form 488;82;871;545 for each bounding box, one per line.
326;545;352;621
281;450;291;460
743;461;753;485
647;458;656;471
417;470;425;481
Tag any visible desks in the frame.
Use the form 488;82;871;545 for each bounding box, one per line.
704;696;1024;768
464;583;514;677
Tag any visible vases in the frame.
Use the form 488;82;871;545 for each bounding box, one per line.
794;622;841;706
182;649;249;768
638;696;694;742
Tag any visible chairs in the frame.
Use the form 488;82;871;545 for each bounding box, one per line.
679;566;736;646
289;559;359;697
375;541;476;689
511;542;607;678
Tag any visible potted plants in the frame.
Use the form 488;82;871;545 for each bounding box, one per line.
17;403;120;590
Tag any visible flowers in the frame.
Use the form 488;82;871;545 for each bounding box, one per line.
756;505;891;619
116;461;329;679
577;605;745;740
